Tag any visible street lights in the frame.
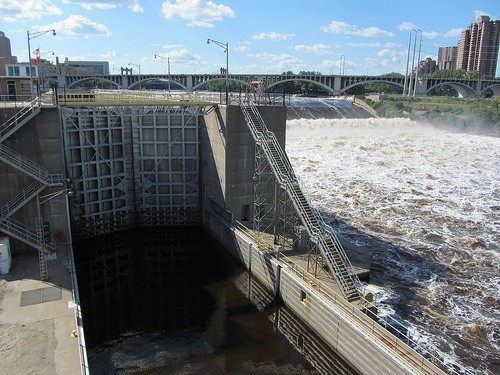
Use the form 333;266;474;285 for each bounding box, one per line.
207;38;229;104
127;62;142;92
38;51;54;90
27;29;57;104
155;54;171;94
